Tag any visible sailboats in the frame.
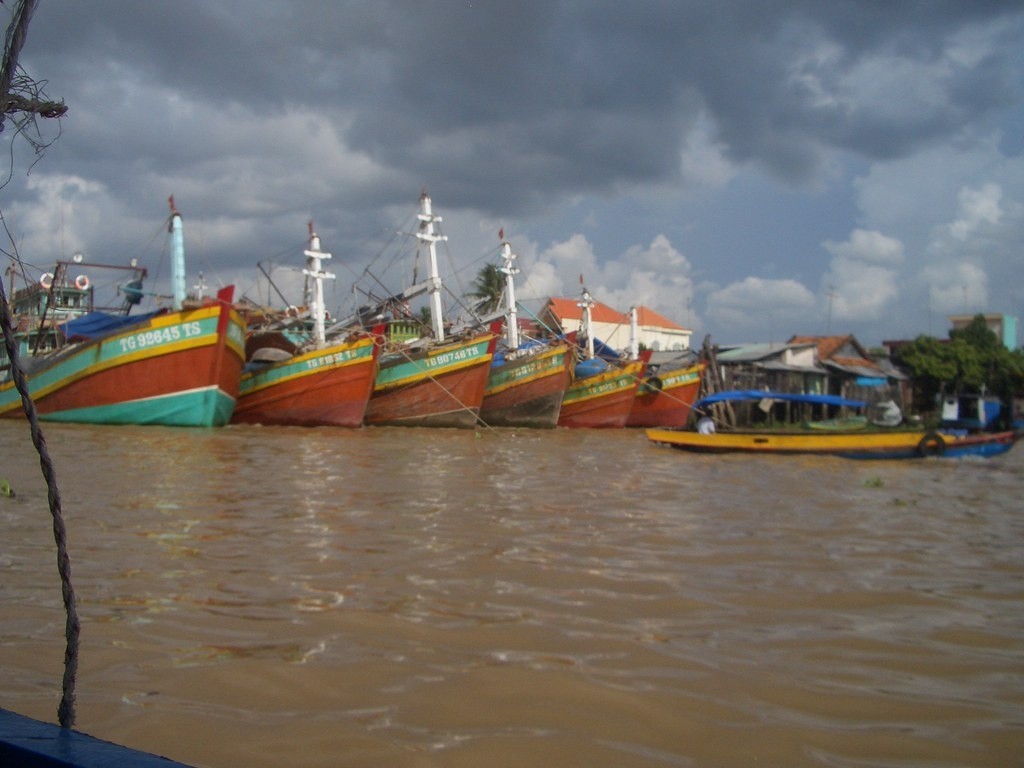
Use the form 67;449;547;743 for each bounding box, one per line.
0;186;709;429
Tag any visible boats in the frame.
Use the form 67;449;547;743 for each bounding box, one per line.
800;414;869;433
646;386;1020;461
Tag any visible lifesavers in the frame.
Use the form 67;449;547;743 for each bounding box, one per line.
40;273;55;289
918;433;945;457
75;275;89;290
285;306;300;318
325;310;332;318
646;376;662;395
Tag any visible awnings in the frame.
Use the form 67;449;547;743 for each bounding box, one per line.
751;357;909;380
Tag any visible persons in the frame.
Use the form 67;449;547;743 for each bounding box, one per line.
696;410;716;434
